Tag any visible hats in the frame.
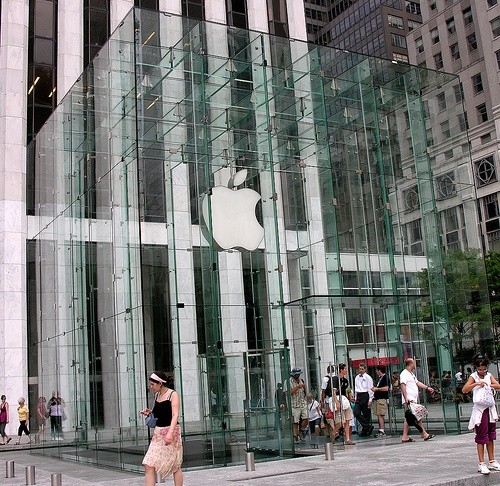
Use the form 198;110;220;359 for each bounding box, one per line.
290;368;303;375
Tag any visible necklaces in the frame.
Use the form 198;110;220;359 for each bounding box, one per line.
158;389;168;395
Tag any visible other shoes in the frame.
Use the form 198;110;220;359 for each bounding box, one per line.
367;426;374;436
359;433;366;437
344;440;356;445
14;442;20;445
0;443;5;445
334;432;340;439
374;430;386;438
487;460;500;471
299;431;306;441
27;440;33;444
477;462;491;474
7;438;12;444
294;434;300;443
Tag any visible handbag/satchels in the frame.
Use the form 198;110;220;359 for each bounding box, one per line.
145;392;158;428
472;383;496;407
405;402;428;433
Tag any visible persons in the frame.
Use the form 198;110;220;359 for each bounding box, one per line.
0;395;12;445
14;397;33;445
462;353;500;474
275;358;473;445
36;397;48;435
47;391;66;441
141;371;183;486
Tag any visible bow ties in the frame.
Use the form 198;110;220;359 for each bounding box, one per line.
359;374;363;377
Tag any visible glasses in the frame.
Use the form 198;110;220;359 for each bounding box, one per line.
477;368;487;373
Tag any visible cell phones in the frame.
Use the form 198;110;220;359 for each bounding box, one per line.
139;410;148;414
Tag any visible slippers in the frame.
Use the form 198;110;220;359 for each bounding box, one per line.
424;433;436;441
402;437;415;442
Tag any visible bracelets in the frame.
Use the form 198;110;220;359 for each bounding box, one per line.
427;386;429;390
